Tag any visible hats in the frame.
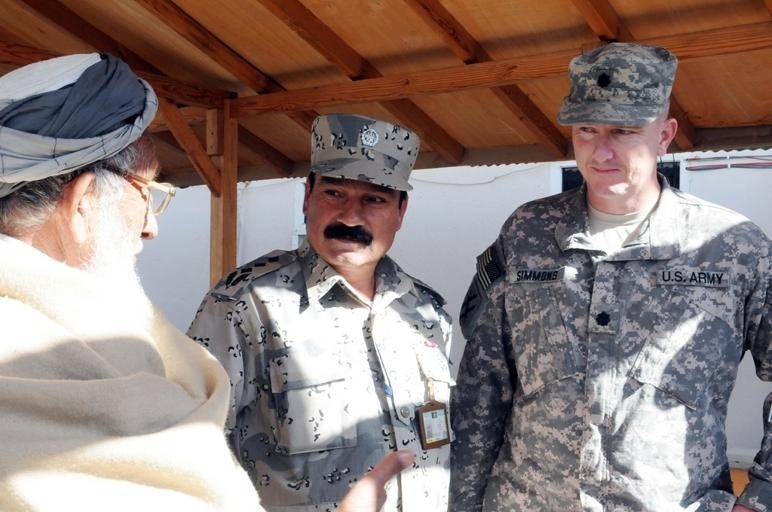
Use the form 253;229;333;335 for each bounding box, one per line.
558;42;678;126
0;52;159;198
310;114;420;191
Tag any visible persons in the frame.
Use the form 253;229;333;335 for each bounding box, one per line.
183;111;458;511
446;40;771;510
0;49;415;511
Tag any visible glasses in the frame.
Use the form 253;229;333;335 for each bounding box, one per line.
98;161;176;216
